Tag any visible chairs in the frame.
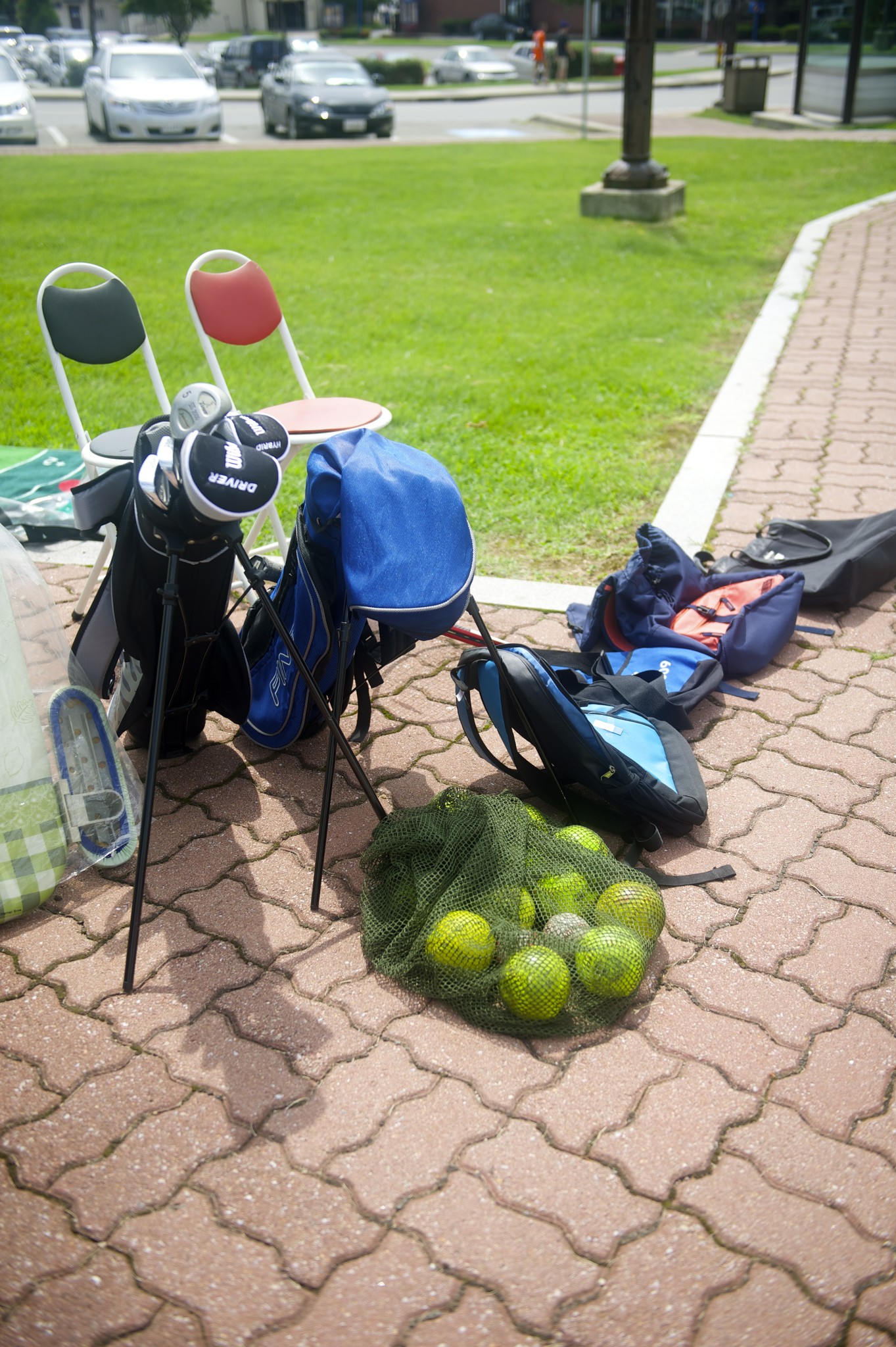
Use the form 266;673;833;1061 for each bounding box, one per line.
183;248;392;602
36;263;259;620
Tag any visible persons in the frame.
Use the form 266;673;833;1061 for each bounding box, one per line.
532;22;549;84
554;20;576;81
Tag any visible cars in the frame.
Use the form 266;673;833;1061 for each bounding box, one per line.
258;51;398;139
0;24;151;81
433;39;562;86
0;47;41;145
82;41;224;142
200;40;232;67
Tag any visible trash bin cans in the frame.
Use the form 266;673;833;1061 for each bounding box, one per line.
720;54;771;114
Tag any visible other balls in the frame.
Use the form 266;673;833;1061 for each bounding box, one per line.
422;791;669;1021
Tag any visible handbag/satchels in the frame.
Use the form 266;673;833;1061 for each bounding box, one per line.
694;506;896;613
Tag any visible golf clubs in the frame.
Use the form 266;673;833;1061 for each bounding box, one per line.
136;381;233;511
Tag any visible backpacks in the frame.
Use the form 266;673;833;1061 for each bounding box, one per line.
565;523;806;681
549;647;724;733
450;643;708;838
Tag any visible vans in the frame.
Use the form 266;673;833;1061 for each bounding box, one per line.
213;35;286;89
